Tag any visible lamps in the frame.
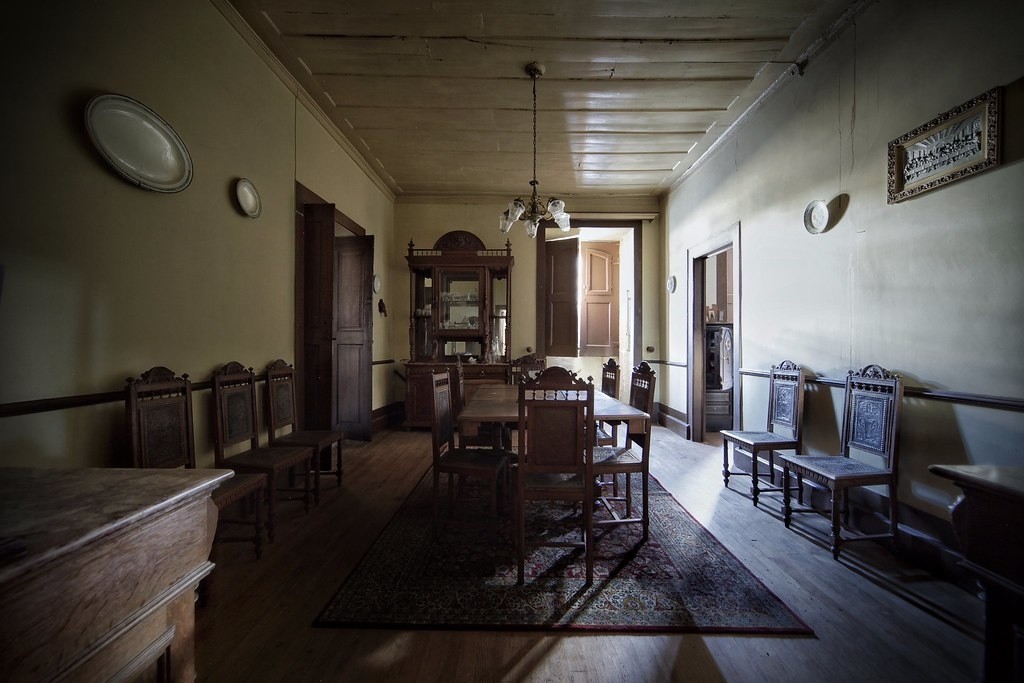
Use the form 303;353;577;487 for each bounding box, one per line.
499;59;570;238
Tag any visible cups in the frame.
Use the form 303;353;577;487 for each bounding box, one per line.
441;288;479;301
439;320;478;329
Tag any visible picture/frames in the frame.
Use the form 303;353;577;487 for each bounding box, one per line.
885;84;1000;205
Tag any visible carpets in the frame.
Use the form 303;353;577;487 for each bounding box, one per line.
309;442;811;632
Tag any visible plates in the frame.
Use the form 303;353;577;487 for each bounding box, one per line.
84;92;193;194
804;200;829;234
235;178;262;219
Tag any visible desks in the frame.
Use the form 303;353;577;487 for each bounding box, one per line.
0;465;236;683
456;383;651;538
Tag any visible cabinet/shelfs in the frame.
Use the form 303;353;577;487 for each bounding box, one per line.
403;237;515;432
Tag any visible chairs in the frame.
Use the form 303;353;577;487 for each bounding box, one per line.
212;360;314;541
427;356;657;587
719;360;804;507
123;366;268;608
265;359;343;507
778;363;903;559
